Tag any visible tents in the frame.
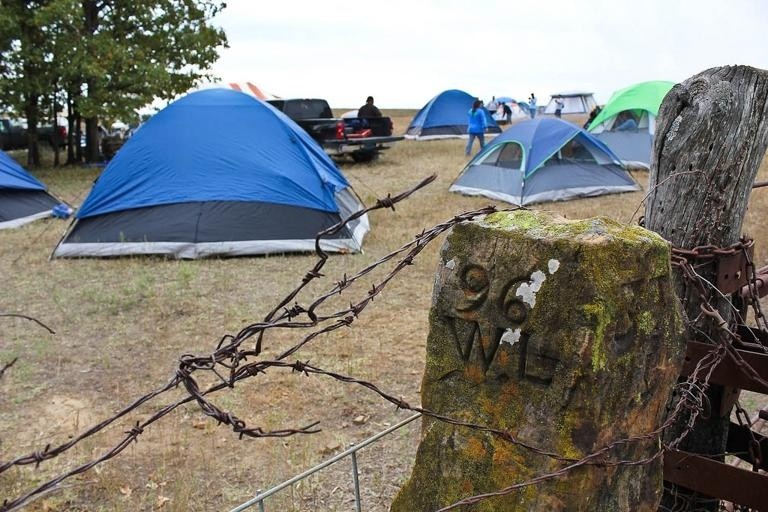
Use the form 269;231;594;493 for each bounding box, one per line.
0;147;72;232
581;79;684;173
543;93;598;114
401;89;504;143
356;95;383;119
47;85;370;260
444;115;643;208
491;102;530;125
485;97;517;110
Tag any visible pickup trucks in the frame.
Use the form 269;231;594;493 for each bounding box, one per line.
1;115;71;154
265;98;407;165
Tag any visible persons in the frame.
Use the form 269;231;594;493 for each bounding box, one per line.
582;111;596;130
528;94;537;119
501;102;512;126
594;105;601;112
553;94;563;118
612;110;639;133
463;101;486;159
487;97;498;116
